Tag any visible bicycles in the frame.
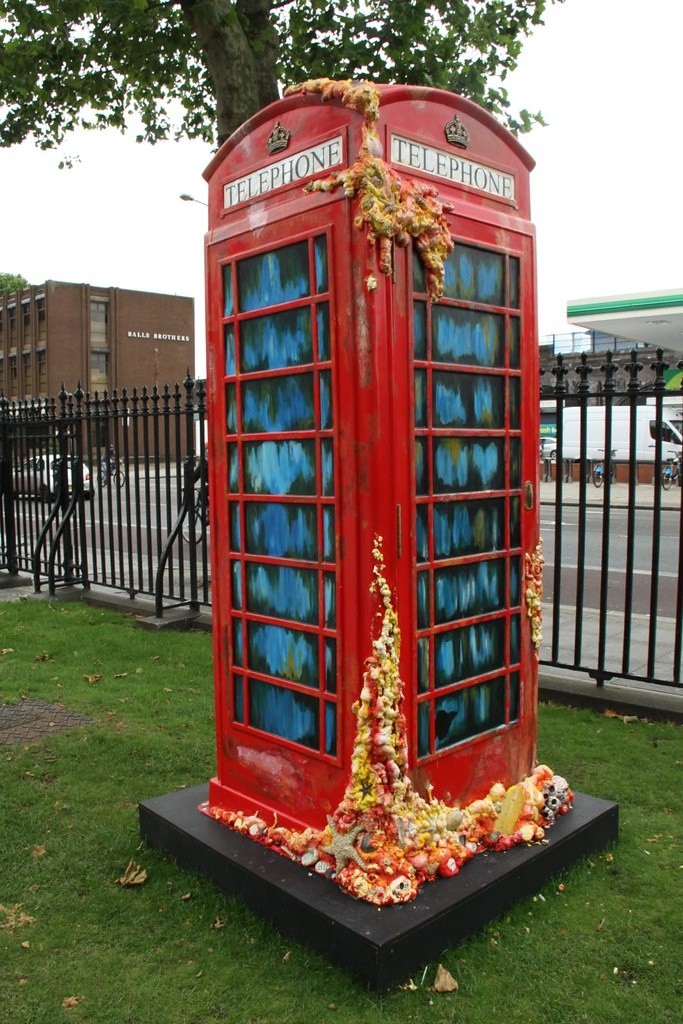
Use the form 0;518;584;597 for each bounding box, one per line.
178;488;212;544
592;448;617;488
97;460;125;488
662;449;683;490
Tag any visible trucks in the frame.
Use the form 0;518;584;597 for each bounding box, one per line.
561;405;683;461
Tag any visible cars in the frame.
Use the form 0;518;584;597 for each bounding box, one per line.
13;454;96;503
538;436;557;461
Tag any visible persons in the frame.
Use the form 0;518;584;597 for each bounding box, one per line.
100;443;116;485
194;442;209;526
